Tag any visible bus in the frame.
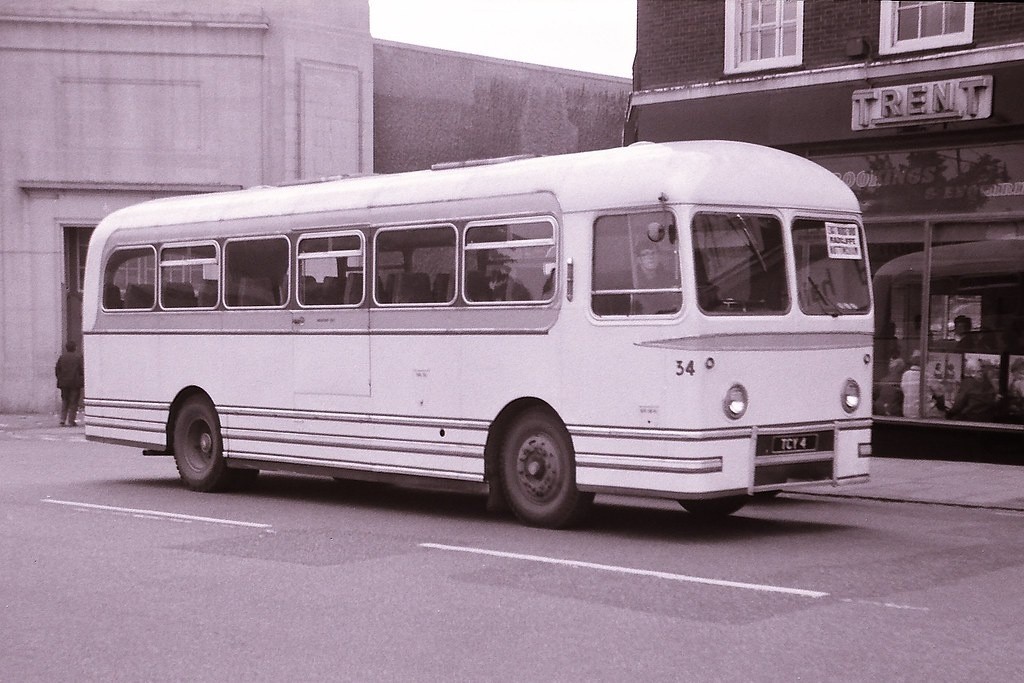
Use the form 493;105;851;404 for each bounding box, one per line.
872;239;1024;418
81;139;877;527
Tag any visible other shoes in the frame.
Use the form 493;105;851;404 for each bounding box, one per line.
68;422;78;426
60;422;65;426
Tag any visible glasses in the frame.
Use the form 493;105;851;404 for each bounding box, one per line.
639;251;658;257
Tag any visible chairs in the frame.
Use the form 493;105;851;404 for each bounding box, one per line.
103;270;507;309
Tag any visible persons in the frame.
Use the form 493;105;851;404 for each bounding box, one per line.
873;315;1024;425
56;342;85;426
